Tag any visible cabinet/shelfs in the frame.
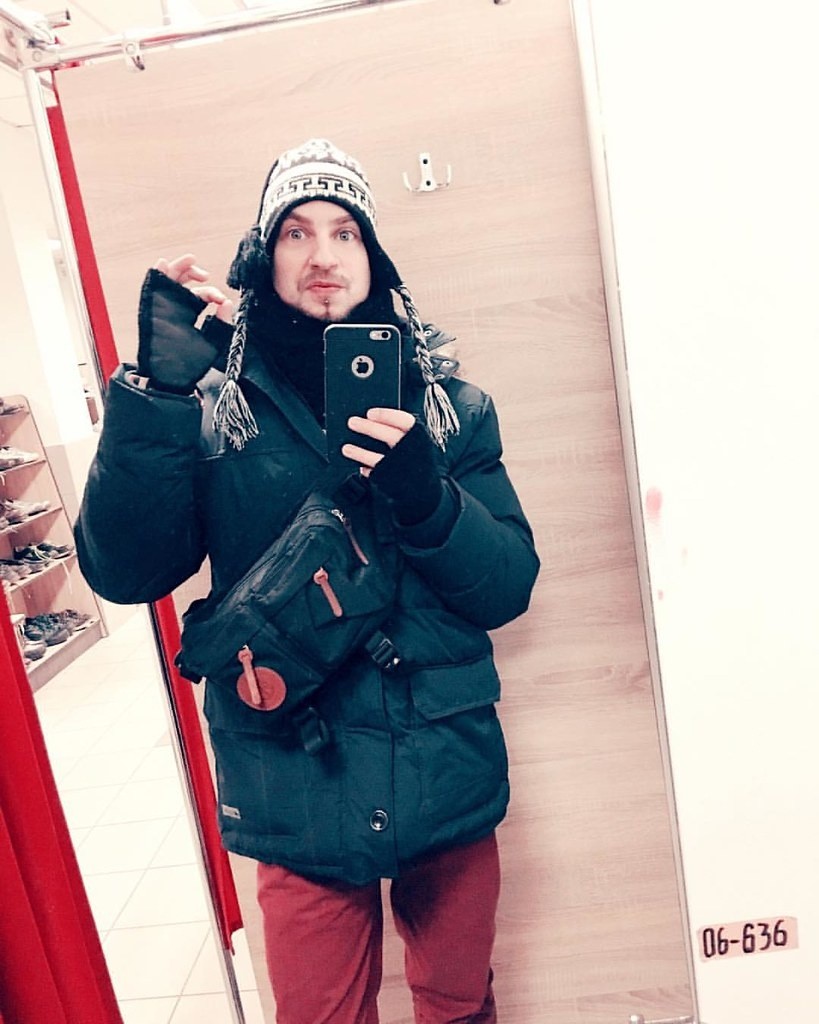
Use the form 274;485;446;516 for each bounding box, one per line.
0;395;108;695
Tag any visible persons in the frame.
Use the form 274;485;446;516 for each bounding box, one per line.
72;136;540;1023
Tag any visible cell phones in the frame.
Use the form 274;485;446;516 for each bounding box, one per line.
323;325;401;467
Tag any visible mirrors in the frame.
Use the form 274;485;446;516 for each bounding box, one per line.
0;0;819;1024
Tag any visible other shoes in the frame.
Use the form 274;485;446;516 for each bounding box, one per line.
0;398;98;666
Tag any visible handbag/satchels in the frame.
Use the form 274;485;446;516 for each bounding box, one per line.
183;495;405;734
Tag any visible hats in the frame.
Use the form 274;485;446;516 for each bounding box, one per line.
213;139;464;454
